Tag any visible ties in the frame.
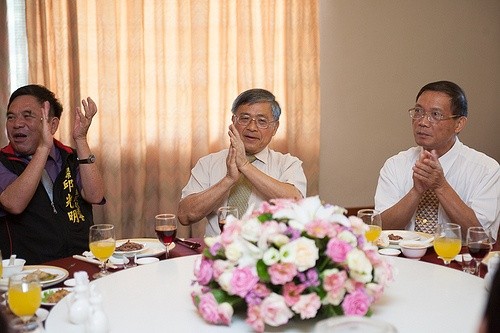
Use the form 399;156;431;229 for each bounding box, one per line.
414;149;441;233
226;154;258;218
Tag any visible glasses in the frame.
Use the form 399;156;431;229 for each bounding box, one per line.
408;103;464;123
236;111;275;130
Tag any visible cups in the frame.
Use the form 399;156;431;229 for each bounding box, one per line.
218;206;238;232
461;255;476;275
9;315;44;333
357;209;381;245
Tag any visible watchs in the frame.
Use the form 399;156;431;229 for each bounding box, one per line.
77;155;96;164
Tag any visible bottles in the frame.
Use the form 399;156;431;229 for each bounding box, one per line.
65;284;111;333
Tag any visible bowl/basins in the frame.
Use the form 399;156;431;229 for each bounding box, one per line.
315;315;398;333
399;239;430;261
2;259;25;278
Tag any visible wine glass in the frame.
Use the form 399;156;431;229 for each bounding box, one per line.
89;224;115;278
433;222;462;268
7;273;42;326
155;213;177;259
466;227;492;278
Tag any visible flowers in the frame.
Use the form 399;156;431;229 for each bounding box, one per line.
192;196;392;333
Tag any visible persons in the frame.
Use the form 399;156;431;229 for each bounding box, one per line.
0;85;106;265
177;89;308;239
368;81;500;248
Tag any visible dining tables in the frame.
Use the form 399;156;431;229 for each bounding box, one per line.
0;236;500;333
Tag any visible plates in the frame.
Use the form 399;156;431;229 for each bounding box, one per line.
481;250;500;266
111;239;175;268
0;266;89;322
378;229;434;255
